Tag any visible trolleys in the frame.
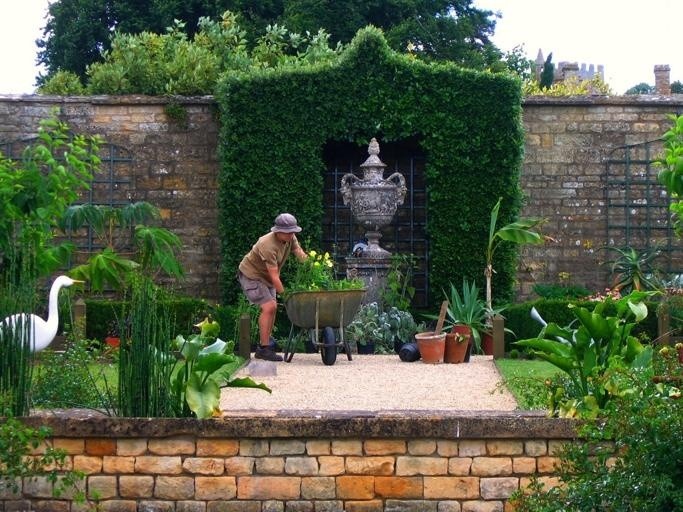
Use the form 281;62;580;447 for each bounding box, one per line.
283;290;365;366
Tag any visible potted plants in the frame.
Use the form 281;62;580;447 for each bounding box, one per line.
105;313;132;346
419;197;559;355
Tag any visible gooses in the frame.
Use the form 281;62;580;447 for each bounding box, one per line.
0;275;86;355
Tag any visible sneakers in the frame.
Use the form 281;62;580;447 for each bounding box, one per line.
255;344;283;361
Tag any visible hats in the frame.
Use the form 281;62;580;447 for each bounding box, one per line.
271;213;302;234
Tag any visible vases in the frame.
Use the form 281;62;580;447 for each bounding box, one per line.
415;331;472;364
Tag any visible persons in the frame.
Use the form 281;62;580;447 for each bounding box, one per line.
238;212;308;361
